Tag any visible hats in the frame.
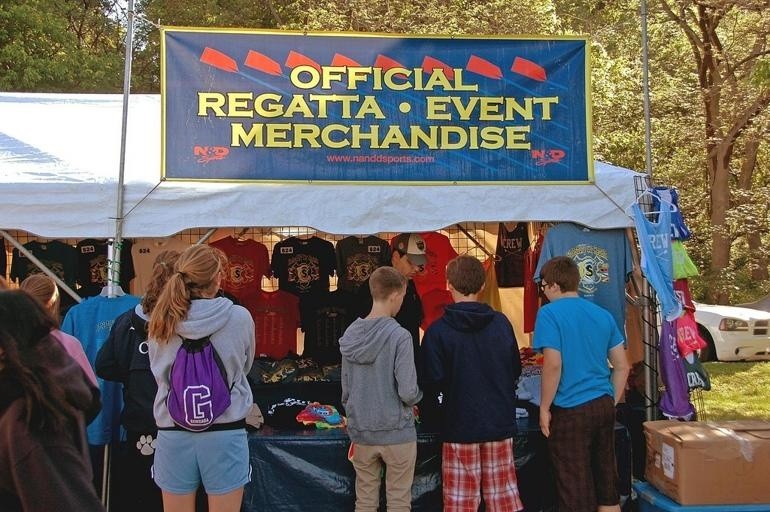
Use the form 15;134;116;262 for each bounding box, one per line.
393;233;428;265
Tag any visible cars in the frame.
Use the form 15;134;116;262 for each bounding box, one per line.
654;290;770;366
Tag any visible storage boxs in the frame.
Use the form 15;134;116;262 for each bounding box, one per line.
632;421;770;512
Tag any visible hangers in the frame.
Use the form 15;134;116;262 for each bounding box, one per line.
66;284;142;322
625;174;678;216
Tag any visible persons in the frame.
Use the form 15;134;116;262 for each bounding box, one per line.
93;248;208;512
19;274;103;434
147;241;257;511
339;265;425;512
531;254;633;512
0;289;105;510
346;233;435;373
420;254;525;512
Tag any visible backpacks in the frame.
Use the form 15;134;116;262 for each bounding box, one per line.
165;332;235;432
123;316;158;434
